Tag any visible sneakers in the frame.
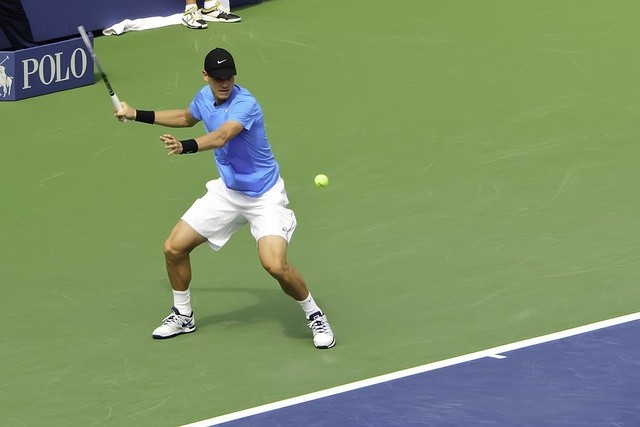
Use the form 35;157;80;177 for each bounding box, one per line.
201;2;241;22
181;6;208;29
152;307;196;339
306;311;336;349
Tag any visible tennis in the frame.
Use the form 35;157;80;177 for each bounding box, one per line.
313;173;327;187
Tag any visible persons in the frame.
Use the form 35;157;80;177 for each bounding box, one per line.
181;0;241;28
114;47;336;350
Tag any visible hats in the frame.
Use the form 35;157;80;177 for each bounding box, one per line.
204;48;237;79
